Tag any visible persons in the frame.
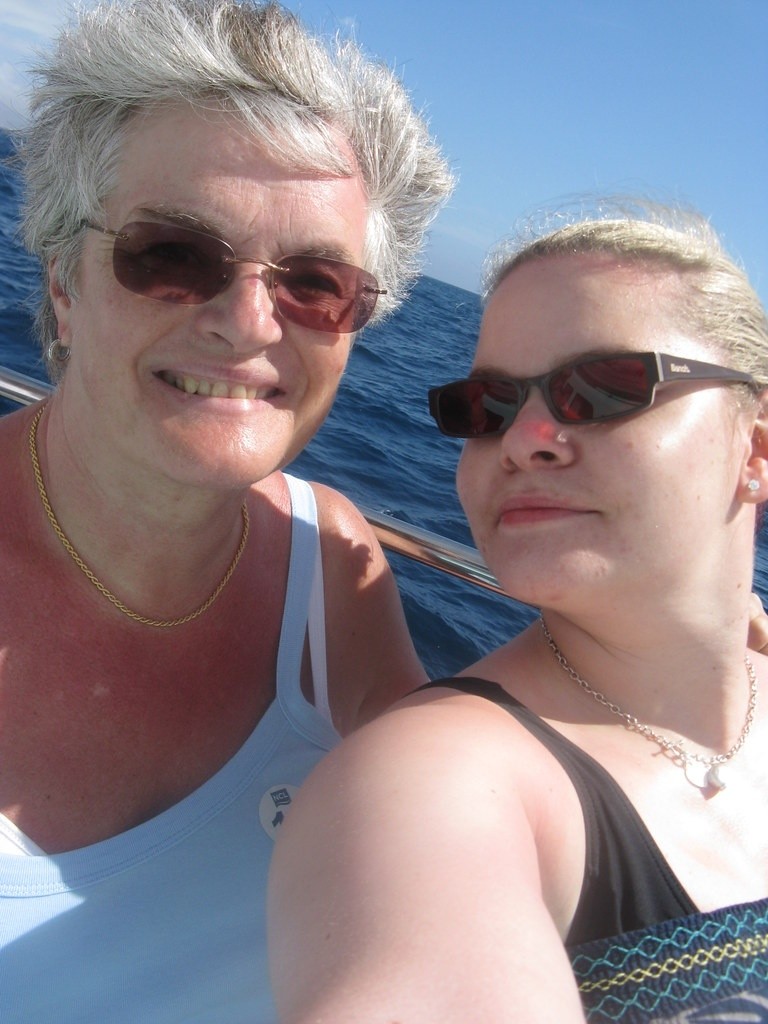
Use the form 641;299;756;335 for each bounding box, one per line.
261;201;765;1022
0;0;766;1024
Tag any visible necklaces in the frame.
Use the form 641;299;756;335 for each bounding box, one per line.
537;607;756;793
29;397;248;631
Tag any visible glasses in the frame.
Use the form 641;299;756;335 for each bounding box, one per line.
428;352;763;438
72;220;388;332
